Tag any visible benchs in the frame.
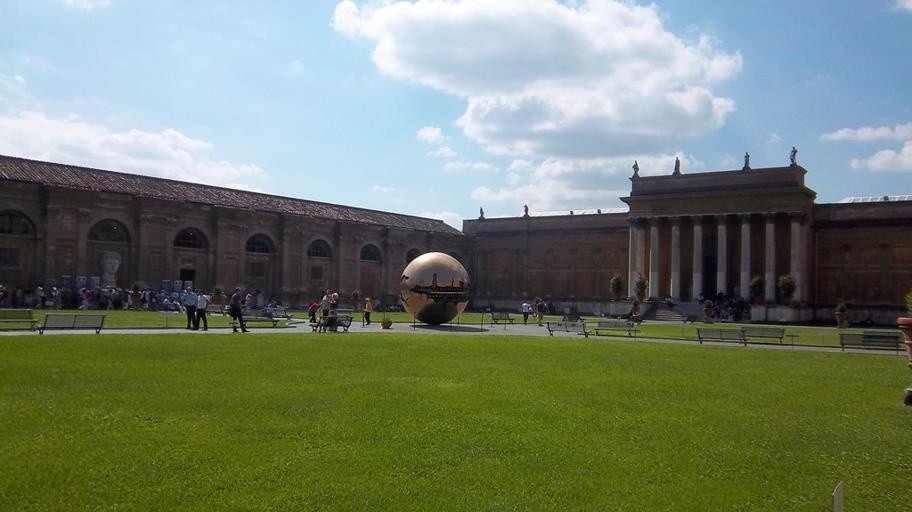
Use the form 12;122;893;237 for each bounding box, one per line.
311;315;353;333
839;330;903;355
697;326;785;346
490;311;514;324
232;309;293;328
0;309;107;334
545;321;641;339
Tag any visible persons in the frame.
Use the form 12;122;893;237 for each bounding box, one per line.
33;285;64;310
628;306;638;324
180;287;211;331
536;298;546;326
635;306;643;325
522;299;530;325
697;289;733;323
78;285;186;311
362;292;374;323
230;287;249;332
666;295;675;306
205;289;227;305
307;288;338;332
0;283;22;308
244;290;281;311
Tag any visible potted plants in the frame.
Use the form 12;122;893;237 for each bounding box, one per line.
895;290;912;369
833;302;846;323
609;272;648;315
750;274;797;306
703;300;713;316
